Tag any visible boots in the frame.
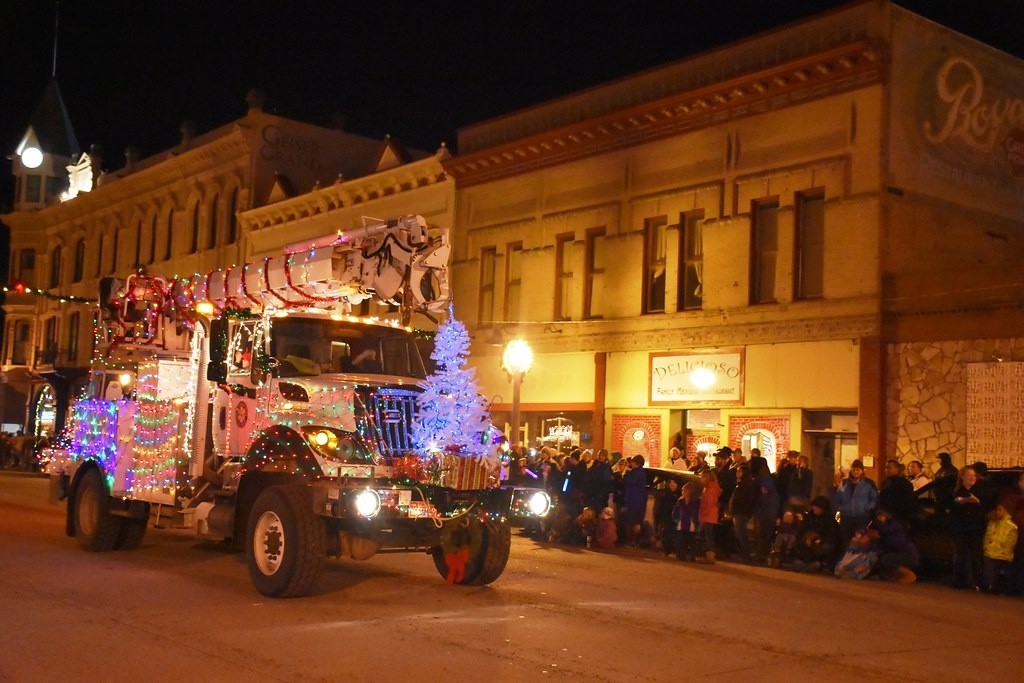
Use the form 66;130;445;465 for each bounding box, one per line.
699;551;715;564
898;565;917;584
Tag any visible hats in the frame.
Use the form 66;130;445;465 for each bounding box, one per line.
571;450;580;462
541;448;551;460
712;452;727;457
716;446;735;453
936;455;941;459
850;459;864;468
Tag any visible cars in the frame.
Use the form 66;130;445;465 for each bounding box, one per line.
642;467;702;543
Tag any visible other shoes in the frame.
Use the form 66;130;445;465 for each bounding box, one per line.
736;559;752;564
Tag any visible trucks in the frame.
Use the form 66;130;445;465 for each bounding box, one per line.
42;213;561;600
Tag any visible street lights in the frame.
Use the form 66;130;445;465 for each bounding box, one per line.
503;337;535;447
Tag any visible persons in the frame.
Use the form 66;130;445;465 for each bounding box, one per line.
999;474;1024;597
933;453;960;505
344;349;383;374
0;429;54;472
949;465;987;593
973;463;986;480
497;432;931;583
983;506;1018;596
